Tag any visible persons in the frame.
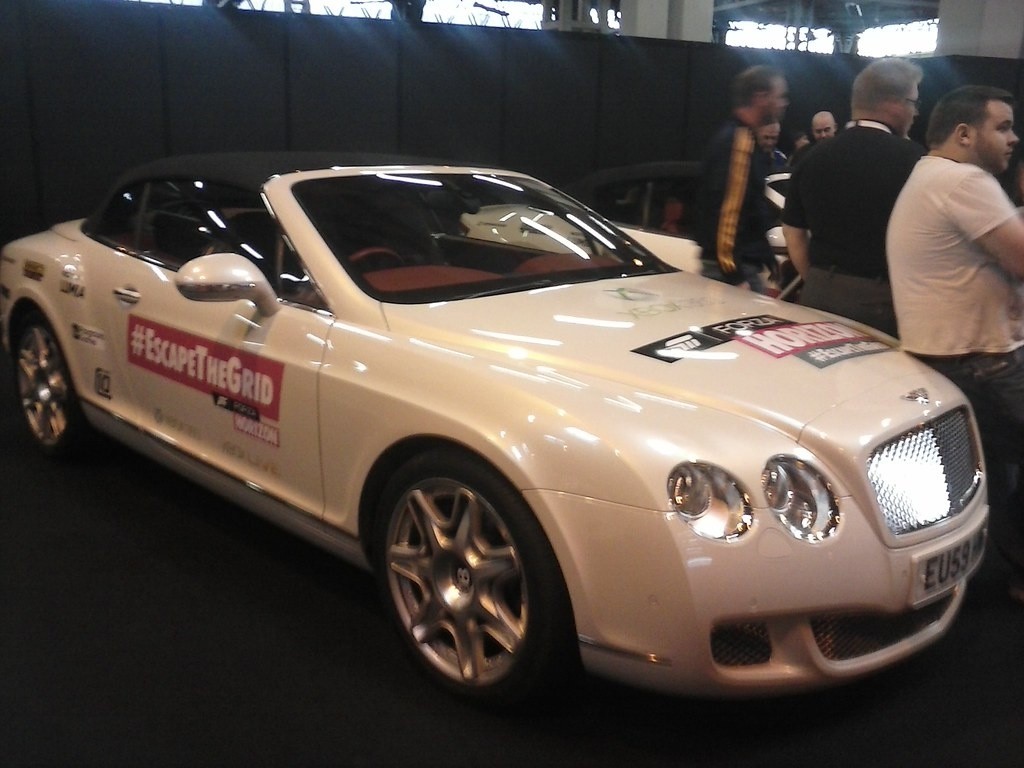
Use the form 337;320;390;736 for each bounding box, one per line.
884;82;1024;605
749;108;839;171
779;57;931;340
685;62;804;308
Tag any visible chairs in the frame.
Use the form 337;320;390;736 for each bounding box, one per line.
203;213;448;288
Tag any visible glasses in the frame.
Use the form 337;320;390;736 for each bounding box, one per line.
885;91;922;109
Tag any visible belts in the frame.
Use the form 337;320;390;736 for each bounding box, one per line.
812;263;882;279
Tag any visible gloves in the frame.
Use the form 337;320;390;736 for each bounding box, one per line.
762;248;782;284
